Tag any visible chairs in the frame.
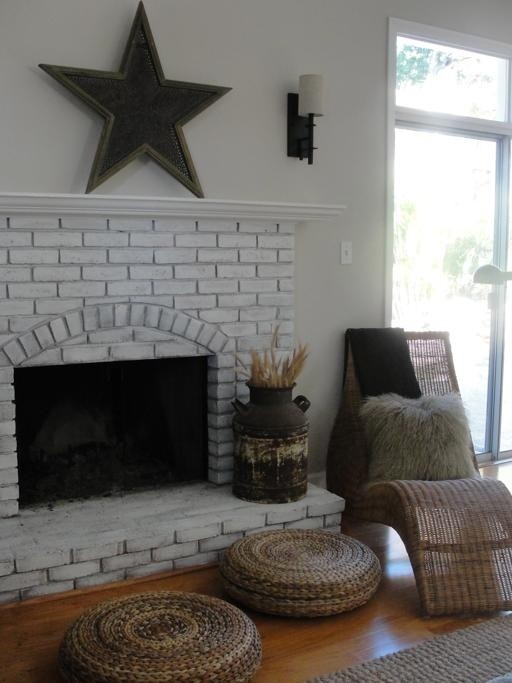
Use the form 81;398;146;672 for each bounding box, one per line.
325;324;512;618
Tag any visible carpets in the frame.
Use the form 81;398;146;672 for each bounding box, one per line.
300;610;510;682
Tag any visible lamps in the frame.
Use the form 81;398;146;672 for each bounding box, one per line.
288;71;324;166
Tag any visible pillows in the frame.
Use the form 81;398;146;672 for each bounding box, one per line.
358;386;481;483
